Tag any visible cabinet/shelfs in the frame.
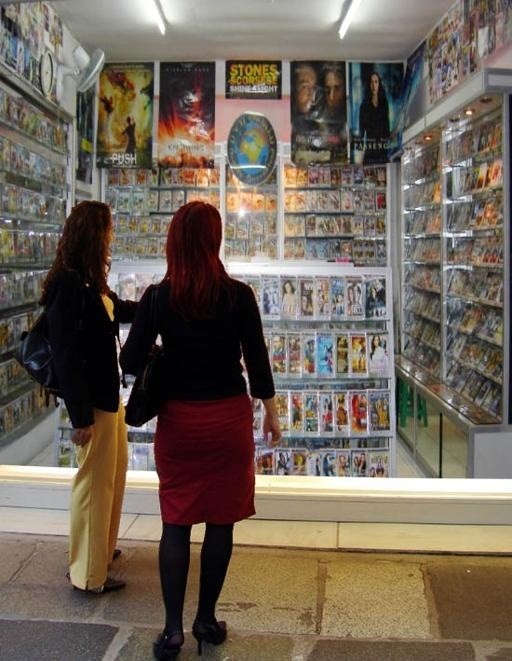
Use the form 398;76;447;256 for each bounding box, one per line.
1;60;75;450
101;168;221;262
280;163;388;266
224;162;279;265
400;68;512;479
59;269;397;476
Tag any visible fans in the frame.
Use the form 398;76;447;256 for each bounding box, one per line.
56;44;105;99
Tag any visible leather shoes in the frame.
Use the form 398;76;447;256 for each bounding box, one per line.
113;548;121;559
66;573;125;593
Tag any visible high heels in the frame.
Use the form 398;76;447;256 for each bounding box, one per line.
153;630;184;661
193;618;226;656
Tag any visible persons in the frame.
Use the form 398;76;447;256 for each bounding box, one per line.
351;70;397;164
290;59;321;141
119;200;282;661
311;63;347;135
38;200;141;594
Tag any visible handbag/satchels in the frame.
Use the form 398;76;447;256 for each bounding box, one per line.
125;350;178;427
14;313;80;395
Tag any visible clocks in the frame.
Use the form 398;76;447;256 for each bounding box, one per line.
41;51;53;95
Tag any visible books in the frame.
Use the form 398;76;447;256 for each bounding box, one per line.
107;270;164;302
237;275;392;477
